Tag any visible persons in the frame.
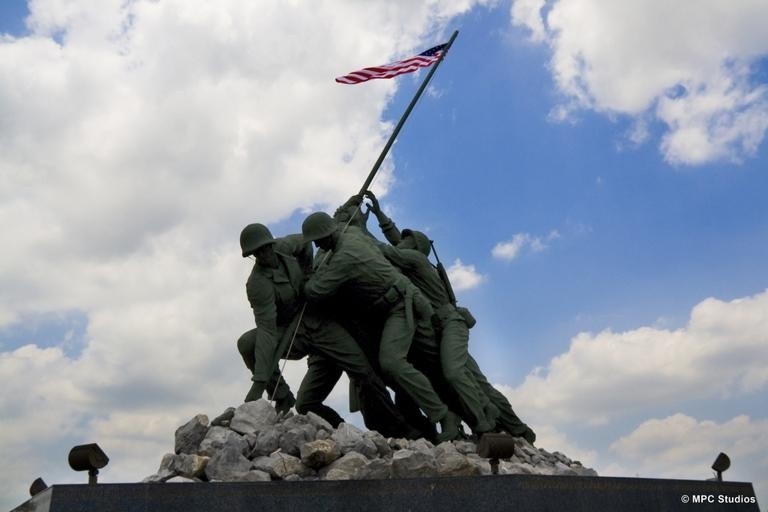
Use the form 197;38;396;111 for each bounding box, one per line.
238;189;536;444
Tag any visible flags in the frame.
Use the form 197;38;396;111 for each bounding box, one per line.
335;43;448;84
